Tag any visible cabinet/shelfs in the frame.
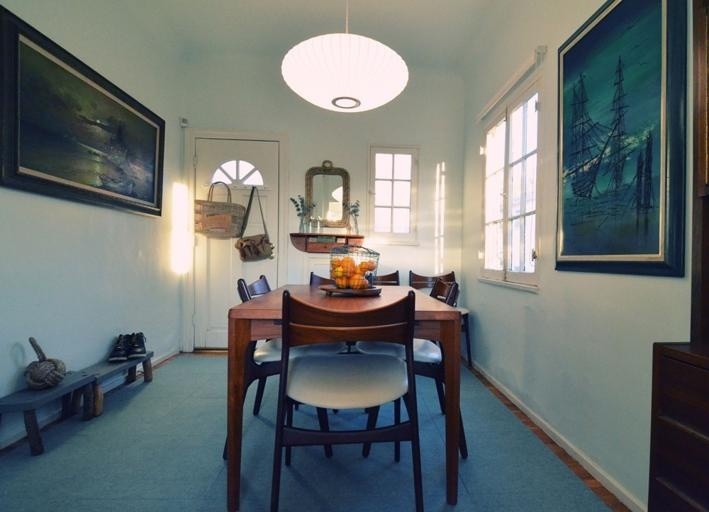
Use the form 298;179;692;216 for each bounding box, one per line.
646;1;708;512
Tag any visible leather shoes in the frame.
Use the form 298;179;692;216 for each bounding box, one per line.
108;334;127;361
128;332;147;359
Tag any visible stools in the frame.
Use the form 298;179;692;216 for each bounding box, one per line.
452;306;472;368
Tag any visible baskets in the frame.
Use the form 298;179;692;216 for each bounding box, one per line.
329;245;380;289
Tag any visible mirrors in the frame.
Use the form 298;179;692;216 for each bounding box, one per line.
305;160;350;227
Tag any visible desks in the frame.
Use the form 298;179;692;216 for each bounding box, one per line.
224;283;461;510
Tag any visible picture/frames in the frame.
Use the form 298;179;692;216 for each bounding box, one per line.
554;0;685;277
0;3;165;220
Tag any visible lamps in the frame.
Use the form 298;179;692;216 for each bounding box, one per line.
280;1;408;114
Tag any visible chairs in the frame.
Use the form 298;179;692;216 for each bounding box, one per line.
409;271;473;364
271;290;425;510
363;278;468;462
223;276;335;459
295;271;400;413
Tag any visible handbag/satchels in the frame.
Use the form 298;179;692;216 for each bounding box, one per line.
236;234;274;262
194;182;246;238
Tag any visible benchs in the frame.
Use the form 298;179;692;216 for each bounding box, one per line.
0;371;96;457
72;352;153;417
